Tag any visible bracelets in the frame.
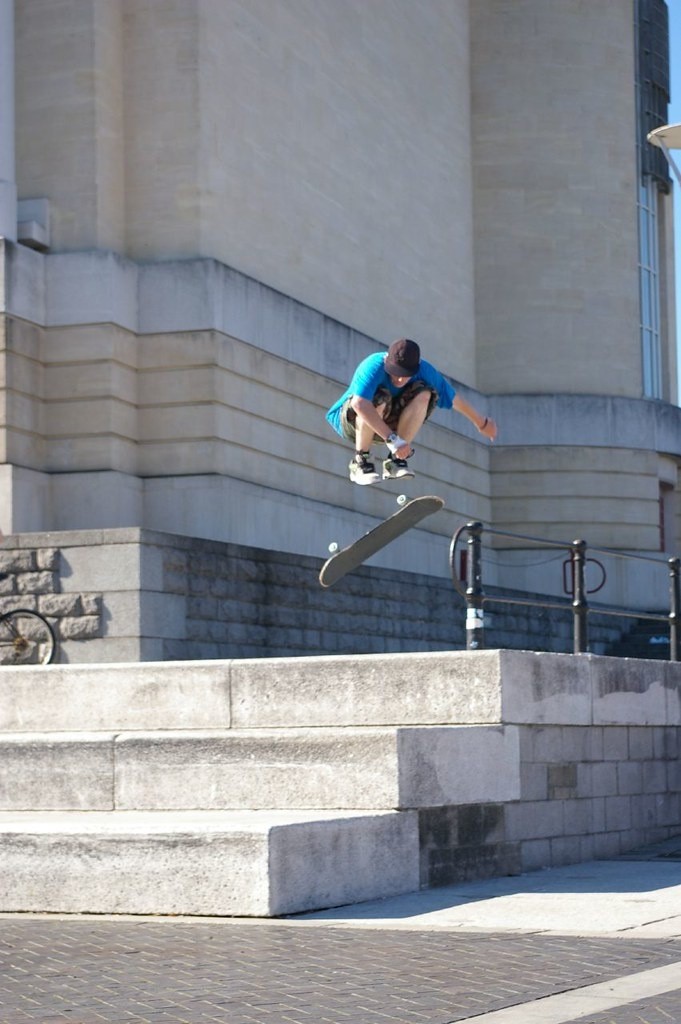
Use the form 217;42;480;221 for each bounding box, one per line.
480;418;488;429
386;432;397;443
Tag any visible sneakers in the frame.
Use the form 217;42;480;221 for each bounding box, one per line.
382;448;415;479
349;449;381;485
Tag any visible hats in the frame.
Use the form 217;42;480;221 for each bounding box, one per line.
385;339;420;376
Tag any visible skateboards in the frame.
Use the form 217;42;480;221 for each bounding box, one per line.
318;492;444;588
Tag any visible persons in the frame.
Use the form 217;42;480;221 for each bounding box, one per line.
324;339;497;486
